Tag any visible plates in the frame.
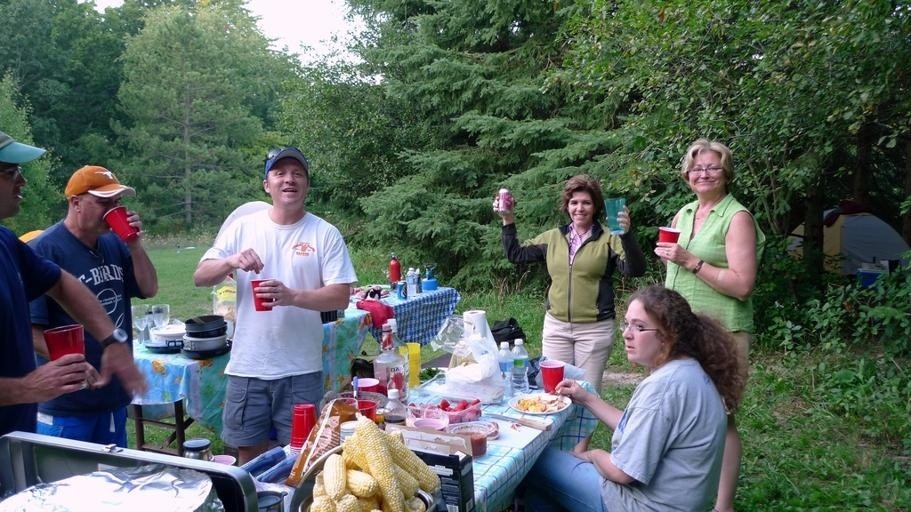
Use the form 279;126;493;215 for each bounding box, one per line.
447;421;496;442
180;313;232;359
507;391;573;416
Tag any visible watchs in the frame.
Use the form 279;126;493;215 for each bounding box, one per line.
99;327;126;347
693;259;704;274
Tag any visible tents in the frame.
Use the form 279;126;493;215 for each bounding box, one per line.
784;207;911;281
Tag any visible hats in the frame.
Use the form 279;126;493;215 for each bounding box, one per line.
65;165;135;198
0;131;46;164
264;145;307;175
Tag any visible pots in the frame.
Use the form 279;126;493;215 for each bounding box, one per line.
146;338;186;354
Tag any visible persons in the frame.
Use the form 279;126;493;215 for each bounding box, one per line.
0;131;148;435
493;175;646;395
25;166;157;450
194;148;358;467
521;287;746;512
654;139;766;371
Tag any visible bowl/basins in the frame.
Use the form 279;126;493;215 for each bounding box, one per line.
398;391;483;423
127;392;188;422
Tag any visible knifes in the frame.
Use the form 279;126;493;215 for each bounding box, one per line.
485;411;554;432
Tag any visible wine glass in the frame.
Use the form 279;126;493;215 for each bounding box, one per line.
127;303;152;352
149;302;173;351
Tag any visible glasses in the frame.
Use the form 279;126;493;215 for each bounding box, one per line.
619;319;660;332
0;165;22;178
690;166;724;174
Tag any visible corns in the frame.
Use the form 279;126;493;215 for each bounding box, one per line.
310;412;441;512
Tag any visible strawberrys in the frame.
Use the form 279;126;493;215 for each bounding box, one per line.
408;398;480;423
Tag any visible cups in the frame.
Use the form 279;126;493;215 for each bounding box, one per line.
358;399;377;425
42;323;90;396
250;279;275;314
289;404;321;450
539;359;566;394
603;196;631;235
657;225;681;257
213;456;237;466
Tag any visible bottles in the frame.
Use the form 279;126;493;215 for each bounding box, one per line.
389;252;401;289
498;188;514;215
406;267;418;297
511;337;530;395
498;339;514;407
182;438;215;462
383;390;407;428
373;323;406;403
396;281;408;302
385;318;409;393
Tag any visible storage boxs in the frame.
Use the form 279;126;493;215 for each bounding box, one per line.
2;431;259;512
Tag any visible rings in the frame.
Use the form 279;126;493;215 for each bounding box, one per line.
665;251;668;255
